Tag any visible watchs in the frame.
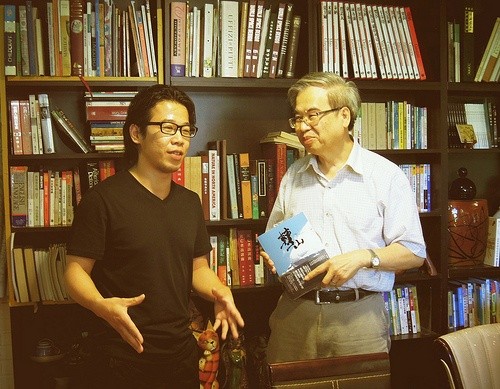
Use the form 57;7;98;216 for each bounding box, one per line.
365;249;380;269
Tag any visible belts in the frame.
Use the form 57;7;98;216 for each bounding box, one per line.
300;288;375;305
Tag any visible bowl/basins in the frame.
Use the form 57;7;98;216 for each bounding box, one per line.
32;338;61;356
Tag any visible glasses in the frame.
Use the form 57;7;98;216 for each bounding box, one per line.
147;120;198;138
288;106;341;128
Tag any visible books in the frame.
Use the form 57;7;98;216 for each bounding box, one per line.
173;130;305;286
0;0;500;82
382;284;421;336
398;164;430;213
483;211;500;267
257;210;330;301
354;94;500;150
8;91;141;303
447;276;500;327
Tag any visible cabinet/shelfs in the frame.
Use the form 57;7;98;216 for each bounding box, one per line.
0;0;500;389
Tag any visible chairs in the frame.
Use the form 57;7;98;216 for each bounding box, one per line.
268;352;391;389
434;323;500;389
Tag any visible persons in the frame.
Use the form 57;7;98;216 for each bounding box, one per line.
260;72;427;364
65;83;245;389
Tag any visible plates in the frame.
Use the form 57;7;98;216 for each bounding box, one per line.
28;353;65;363
